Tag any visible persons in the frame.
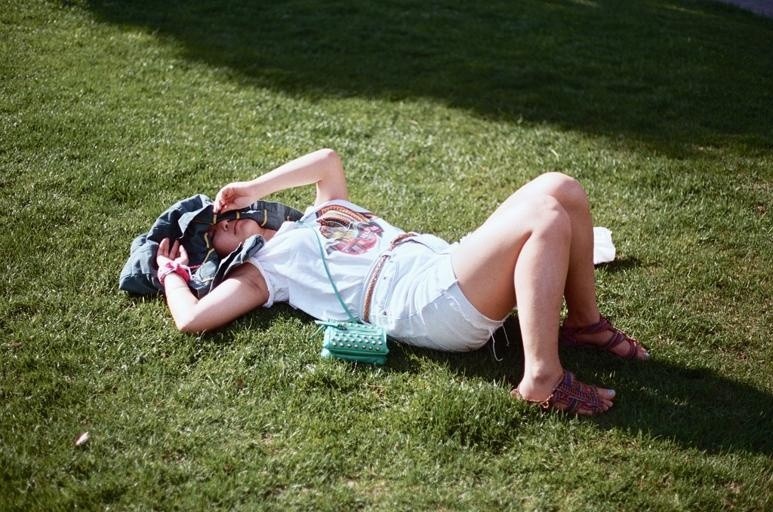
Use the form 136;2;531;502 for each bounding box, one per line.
157;148;650;416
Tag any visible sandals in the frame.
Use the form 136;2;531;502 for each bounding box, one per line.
508;369;616;417
559;313;650;362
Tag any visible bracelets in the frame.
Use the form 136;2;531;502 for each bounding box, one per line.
157;263;189;286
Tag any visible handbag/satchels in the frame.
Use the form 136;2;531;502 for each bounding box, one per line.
321;322;390;366
